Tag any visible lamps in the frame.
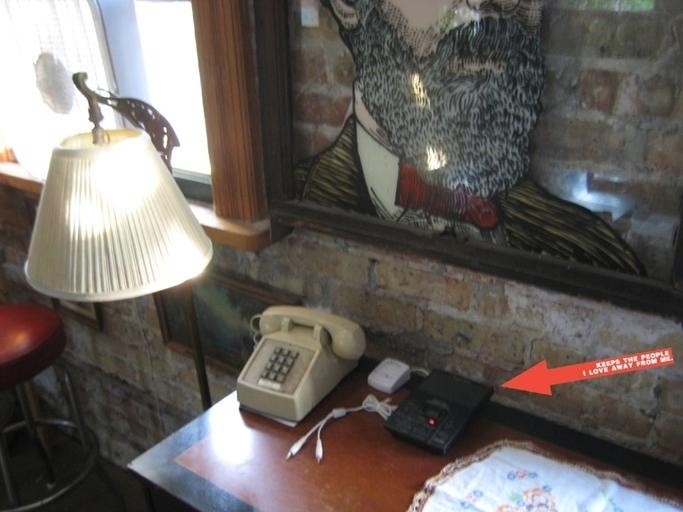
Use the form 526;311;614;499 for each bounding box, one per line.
21;69;213;413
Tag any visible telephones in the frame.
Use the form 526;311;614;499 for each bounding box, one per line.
235;304;368;427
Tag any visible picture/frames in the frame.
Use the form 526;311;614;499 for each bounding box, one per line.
251;2;682;319
25;195;102;331
152;263;304;378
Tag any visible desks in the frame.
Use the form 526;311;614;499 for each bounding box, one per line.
126;354;683;511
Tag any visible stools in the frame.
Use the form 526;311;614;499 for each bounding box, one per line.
0;303;102;511
